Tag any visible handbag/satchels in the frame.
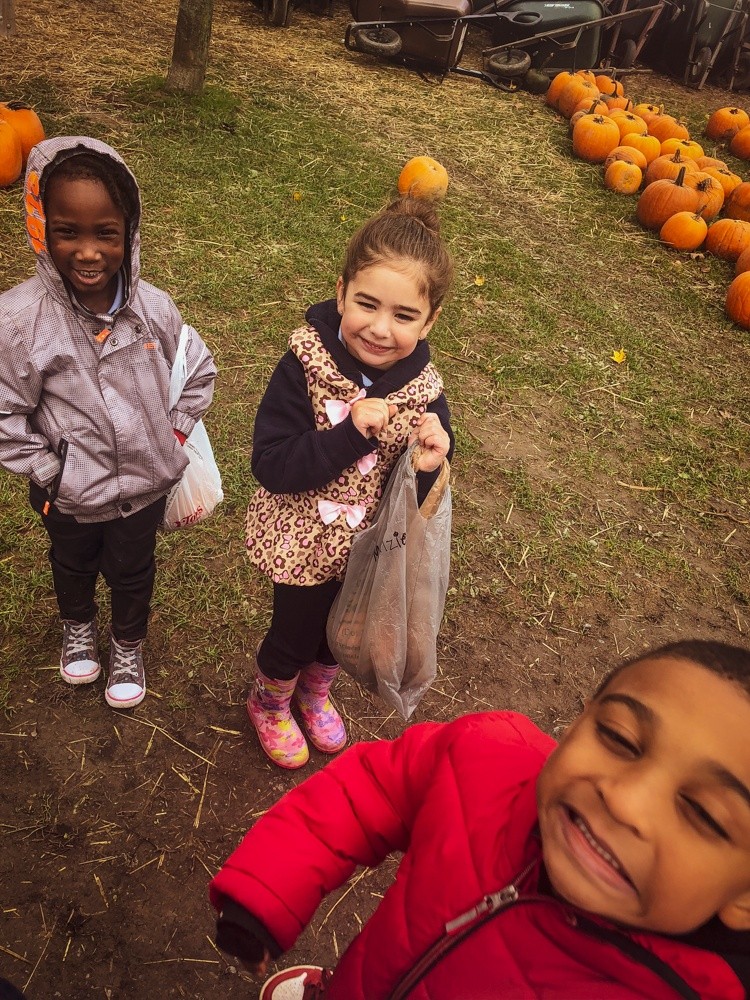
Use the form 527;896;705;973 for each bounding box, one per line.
163;325;225;530
326;441;455;726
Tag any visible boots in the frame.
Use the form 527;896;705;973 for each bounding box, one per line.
295;660;347;753
247;641;309;769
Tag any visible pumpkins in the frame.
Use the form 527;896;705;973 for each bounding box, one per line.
398;156;450;199
0;100;45;187
546;68;750;328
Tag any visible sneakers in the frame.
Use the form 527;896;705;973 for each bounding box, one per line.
257;966;335;1000
105;628;147;709
60;616;101;684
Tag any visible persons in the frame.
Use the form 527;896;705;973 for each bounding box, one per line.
248;198;456;770
217;635;750;999
0;135;218;711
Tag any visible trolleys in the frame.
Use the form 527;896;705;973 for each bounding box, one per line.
481;0;663;77
603;0;750;90
344;0;542;93
